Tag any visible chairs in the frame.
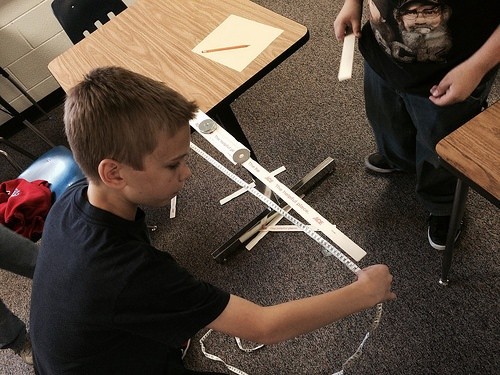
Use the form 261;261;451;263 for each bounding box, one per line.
51;0;128;45
15;144;83;233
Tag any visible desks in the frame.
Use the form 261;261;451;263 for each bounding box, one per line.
436;98;500;285
48;0;336;262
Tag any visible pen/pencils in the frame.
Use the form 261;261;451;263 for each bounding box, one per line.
200;44;250;54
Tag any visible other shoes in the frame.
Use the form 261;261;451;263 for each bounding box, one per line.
13;332;33;366
427;212;463;251
365;152;402;173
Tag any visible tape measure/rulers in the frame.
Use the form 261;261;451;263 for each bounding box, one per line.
189;142;383;375
189;108;367;262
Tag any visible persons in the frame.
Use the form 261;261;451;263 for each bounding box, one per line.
334;0;500;250
0;223;40;364
30;68;396;375
